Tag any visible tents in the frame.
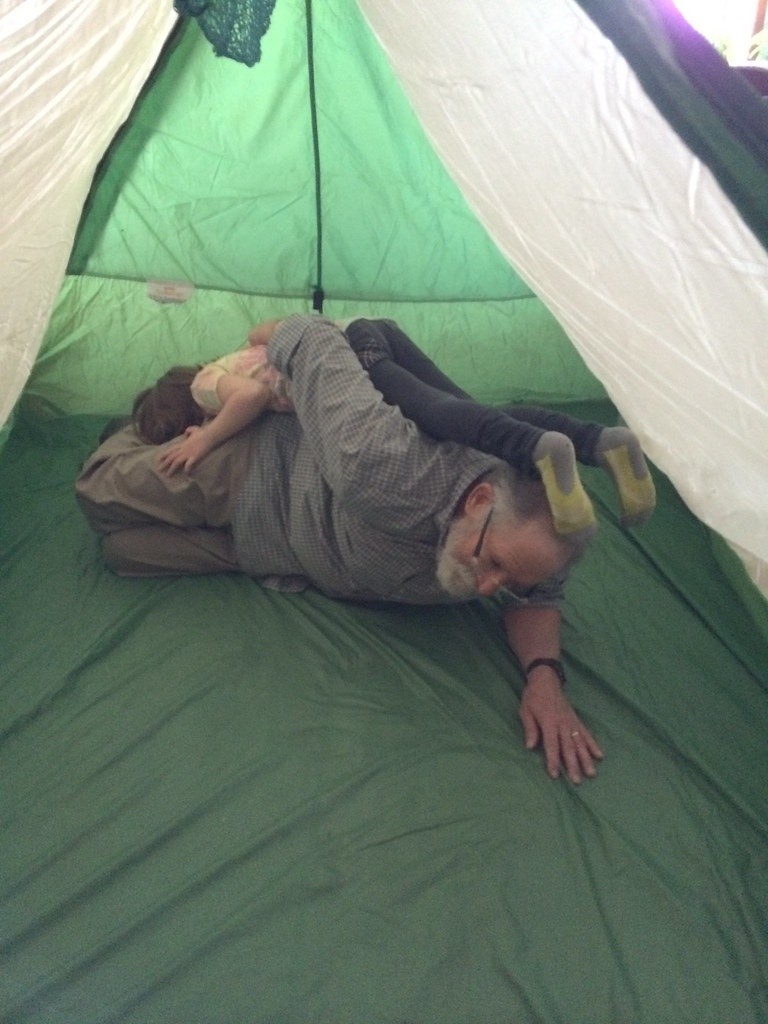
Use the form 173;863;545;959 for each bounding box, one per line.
0;0;768;1024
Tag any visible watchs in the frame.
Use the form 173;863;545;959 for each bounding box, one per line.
527;658;566;685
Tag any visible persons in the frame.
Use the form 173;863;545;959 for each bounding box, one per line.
132;318;655;543
73;313;603;782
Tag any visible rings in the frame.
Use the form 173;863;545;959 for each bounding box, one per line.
572;732;579;736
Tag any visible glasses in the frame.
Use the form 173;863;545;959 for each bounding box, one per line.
468;508;531;612
